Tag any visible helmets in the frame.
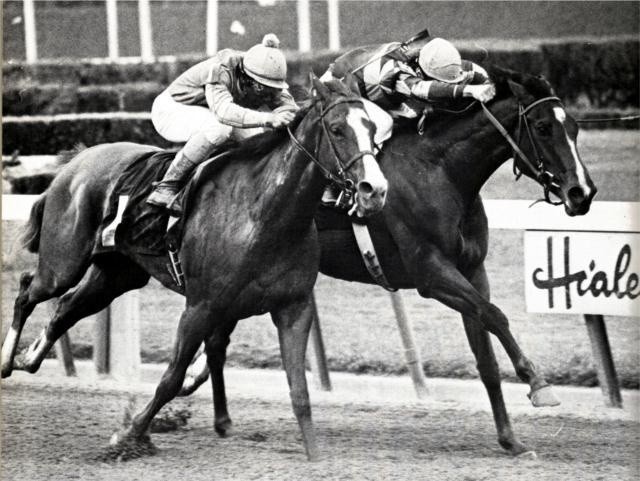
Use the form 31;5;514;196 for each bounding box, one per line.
244;33;290;89
419;37;468;83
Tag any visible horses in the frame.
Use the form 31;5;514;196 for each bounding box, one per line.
1;62;389;467
175;70;598;461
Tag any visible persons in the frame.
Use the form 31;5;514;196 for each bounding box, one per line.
311;32;497;158
144;31;299;217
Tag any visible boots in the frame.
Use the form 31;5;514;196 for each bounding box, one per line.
147;151;198;216
